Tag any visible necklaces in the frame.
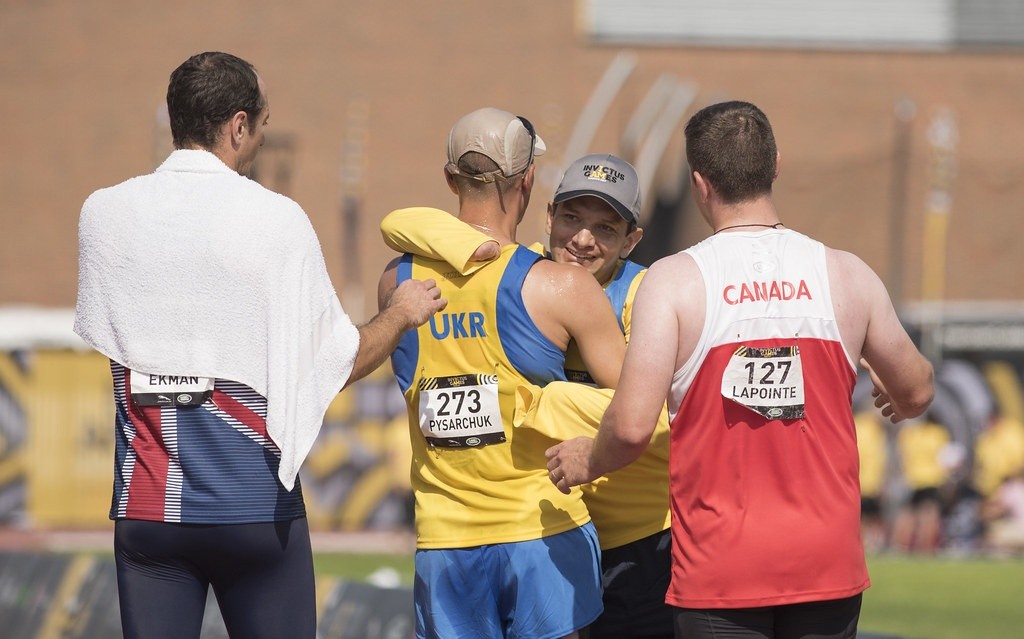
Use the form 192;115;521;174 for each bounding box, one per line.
713;222;784;235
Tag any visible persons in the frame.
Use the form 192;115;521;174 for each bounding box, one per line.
378;107;625;639
379;153;677;639
73;52;446;639
854;358;1024;553
545;100;937;639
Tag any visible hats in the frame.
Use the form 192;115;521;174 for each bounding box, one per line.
553;154;641;227
445;107;546;184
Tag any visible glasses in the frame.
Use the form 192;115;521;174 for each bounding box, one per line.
516;116;534;174
